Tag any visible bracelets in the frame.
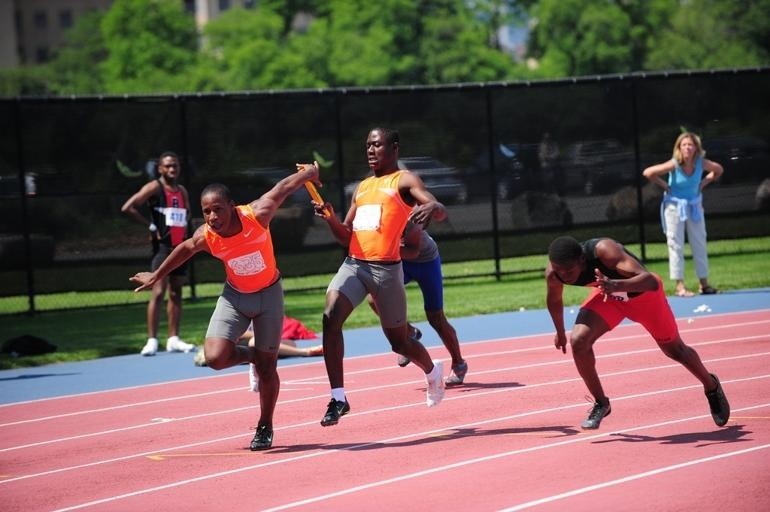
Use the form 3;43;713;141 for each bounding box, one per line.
149;223;157;232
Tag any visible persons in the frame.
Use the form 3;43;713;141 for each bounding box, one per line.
193;313;318;393
129;161;323;451
311;127;448;426
544;236;730;430
121;151;194;356
368;212;468;385
642;131;724;297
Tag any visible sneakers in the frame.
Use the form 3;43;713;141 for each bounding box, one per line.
321;395;350;426
398;328;422;368
166;336;195;352
582;396;611;430
141;338;159;356
250;424;273;451
309;345;323;356
249;364;260;393
423;360;445;407
699;286;720;294
676;287;694;297
445;362;467;386
704;373;729;426
